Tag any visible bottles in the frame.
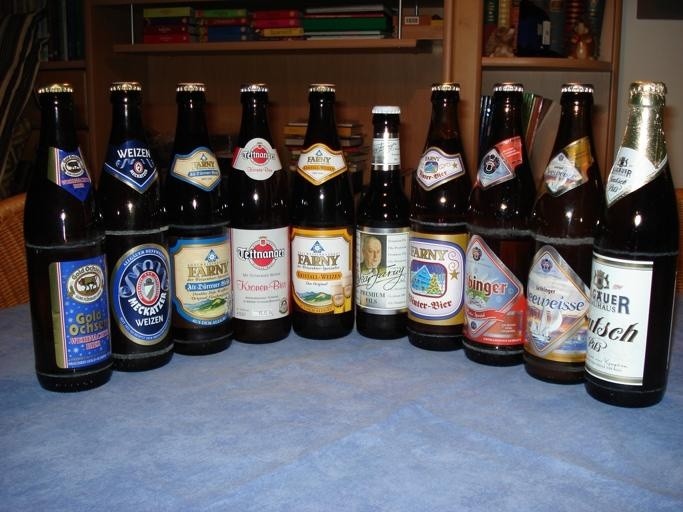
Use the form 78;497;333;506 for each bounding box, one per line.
403;80;469;351
582;80;681;409
103;76;178;374
289;83;353;339
163;76;232;358
353;105;406;339
519;81;606;384
23;80;112;391
458;80;536;363
229;83;292;346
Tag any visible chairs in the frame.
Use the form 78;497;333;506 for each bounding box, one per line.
0;189;30;311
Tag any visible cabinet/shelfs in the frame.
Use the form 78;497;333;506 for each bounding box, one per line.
81;1;452;224
452;0;623;194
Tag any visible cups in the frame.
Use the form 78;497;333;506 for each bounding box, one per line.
566;24;593;59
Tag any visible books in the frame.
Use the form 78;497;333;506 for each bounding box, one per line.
283;115;372;195
141;1;397;42
481;0;603;62
